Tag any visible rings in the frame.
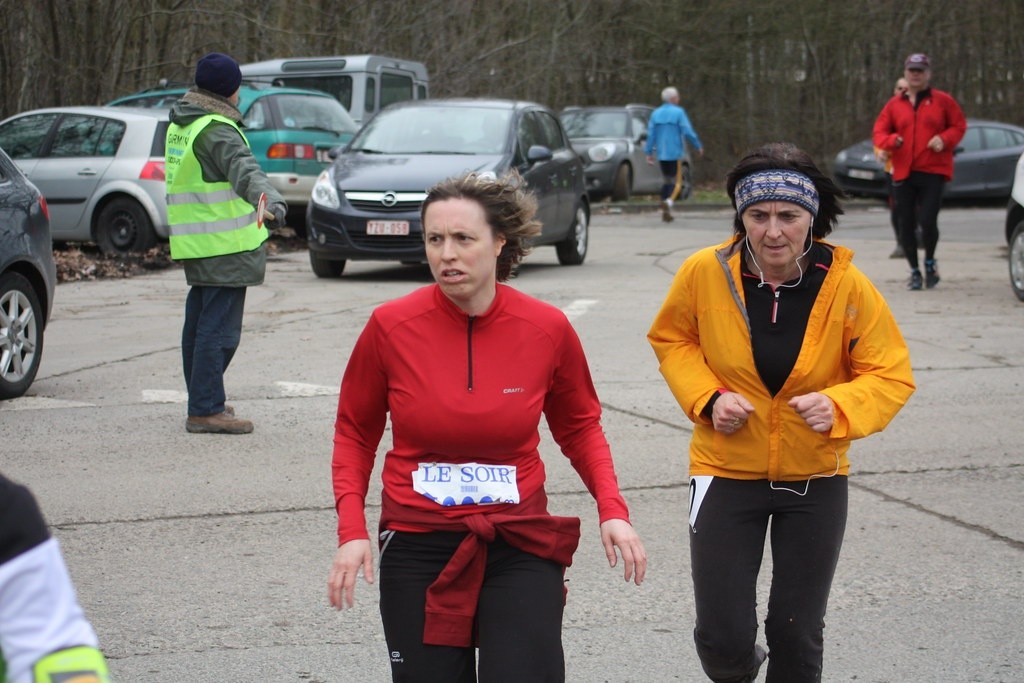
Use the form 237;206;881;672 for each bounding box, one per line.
734;418;739;424
730;423;734;429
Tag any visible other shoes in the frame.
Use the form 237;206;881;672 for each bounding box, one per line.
661;198;674;221
907;270;922;291
890;246;906;258
926;259;939;288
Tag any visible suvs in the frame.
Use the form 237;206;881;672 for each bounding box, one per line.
559;105;691;203
109;80;359;241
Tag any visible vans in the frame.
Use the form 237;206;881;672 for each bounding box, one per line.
243;54;429;130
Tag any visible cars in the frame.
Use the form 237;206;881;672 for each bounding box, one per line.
1004;154;1024;301
834;119;1024;213
306;96;590;277
0;107;170;266
0;149;58;401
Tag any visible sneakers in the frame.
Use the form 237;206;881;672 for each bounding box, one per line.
223;405;235;414
186;411;254;435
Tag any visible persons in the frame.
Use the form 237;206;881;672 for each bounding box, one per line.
648;142;916;683
326;173;648;683
164;53;288;435
645;86;703;222
0;473;109;682
873;54;967;288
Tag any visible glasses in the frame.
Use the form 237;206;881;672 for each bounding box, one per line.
909;68;925;73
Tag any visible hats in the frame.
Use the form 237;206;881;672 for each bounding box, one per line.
196;54;241;97
904;53;930;71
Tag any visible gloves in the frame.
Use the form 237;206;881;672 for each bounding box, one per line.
263;202;287;231
